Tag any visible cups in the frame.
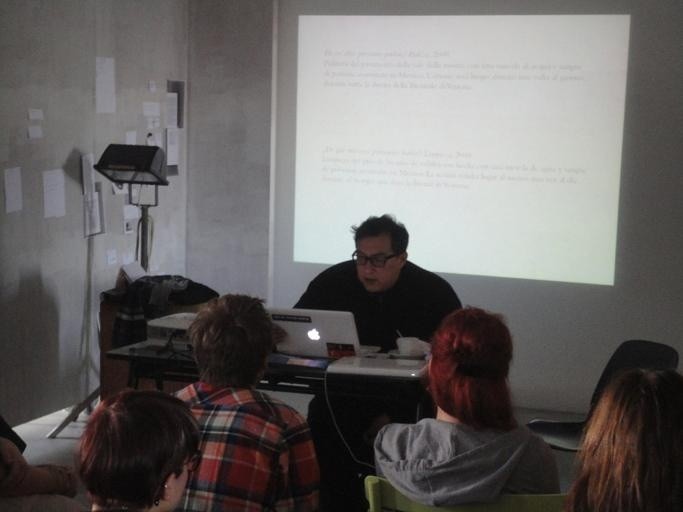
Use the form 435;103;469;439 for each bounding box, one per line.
396;337;419;353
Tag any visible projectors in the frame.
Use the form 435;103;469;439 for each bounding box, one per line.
145;308;204;347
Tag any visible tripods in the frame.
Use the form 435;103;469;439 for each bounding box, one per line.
46;203;156;437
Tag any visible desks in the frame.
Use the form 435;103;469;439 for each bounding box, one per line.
104;344;437;512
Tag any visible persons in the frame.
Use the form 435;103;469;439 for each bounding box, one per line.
77;387;199;510
563;364;682;512
355;306;561;511
165;290;323;511
285;214;469;509
0;413;79;500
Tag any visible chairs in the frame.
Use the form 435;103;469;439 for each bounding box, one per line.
366;475;573;512
525;339;679;453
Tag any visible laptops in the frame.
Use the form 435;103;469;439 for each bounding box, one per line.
266;305;380;364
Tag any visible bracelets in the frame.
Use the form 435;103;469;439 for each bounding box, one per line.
50;464;75;495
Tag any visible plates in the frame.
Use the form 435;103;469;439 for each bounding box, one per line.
390;351;425;358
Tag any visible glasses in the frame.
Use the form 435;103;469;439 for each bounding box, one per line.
183;448;201;470
352;251;396;267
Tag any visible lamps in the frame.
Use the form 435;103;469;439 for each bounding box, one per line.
46;142;169;439
